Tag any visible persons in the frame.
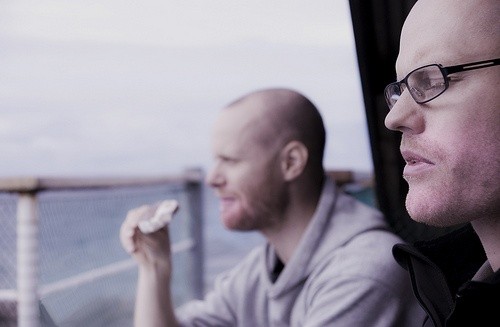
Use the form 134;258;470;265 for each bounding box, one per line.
380;0;500;327
119;87;426;327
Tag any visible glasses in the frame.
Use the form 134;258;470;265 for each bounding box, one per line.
385;57;500;110
